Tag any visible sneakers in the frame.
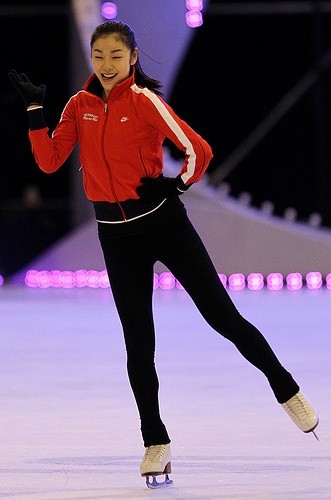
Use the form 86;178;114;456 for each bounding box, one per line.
282;390;320;442
141;443;174;488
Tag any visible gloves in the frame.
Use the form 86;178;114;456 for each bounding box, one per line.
136;174;180;202
8;69;45;105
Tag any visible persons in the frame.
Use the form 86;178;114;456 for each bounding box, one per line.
9;21;321;478
